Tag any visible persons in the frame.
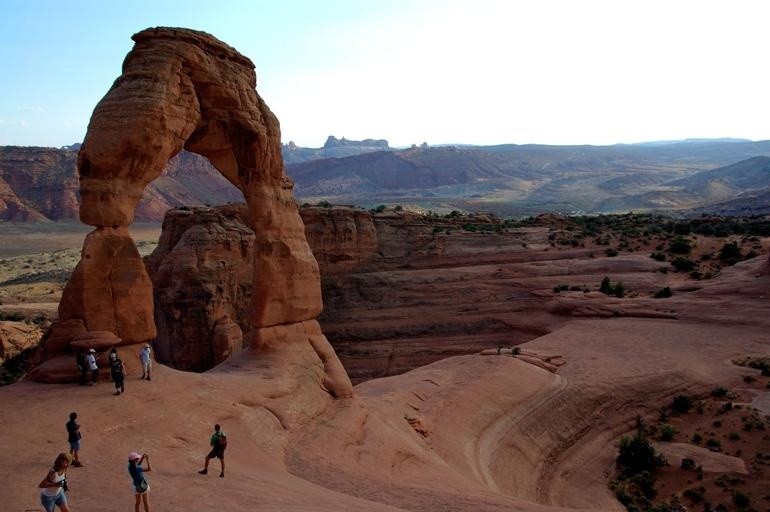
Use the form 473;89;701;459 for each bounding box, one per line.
128;452;152;511
199;424;227;477
76;348;99;385
109;348;126;395
139;343;152;380
66;412;84;467
39;452;71;511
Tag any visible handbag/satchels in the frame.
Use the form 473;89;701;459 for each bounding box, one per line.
137;482;148;493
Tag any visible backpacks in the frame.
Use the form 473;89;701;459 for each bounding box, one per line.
215;432;227;451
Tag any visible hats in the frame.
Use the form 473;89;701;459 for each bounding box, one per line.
128;453;141;461
90;349;97;353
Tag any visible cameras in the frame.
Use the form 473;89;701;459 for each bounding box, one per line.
57;478;70;492
143;454;148;458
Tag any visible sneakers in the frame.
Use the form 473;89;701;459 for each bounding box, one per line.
219;472;224;478
198;469;208;474
